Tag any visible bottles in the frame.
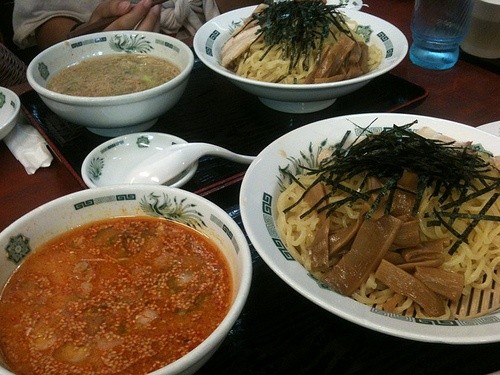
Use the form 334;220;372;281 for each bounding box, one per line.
0;44;34;96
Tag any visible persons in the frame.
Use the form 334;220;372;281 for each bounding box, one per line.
12;0;267;50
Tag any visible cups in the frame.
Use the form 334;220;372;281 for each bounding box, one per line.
409;0;500;70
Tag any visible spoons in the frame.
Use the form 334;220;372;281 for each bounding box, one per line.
138;142;256;184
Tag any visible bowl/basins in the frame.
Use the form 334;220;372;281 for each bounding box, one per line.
192;5;409;114
0;86;21;141
26;28;194;138
0;183;252;375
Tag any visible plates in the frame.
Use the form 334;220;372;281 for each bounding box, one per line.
80;131;199;188
239;111;500;344
273;0;364;10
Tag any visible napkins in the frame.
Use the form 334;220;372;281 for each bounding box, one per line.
3;118;53;175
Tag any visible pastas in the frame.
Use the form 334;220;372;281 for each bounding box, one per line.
237;19;383;84
276;168;500;320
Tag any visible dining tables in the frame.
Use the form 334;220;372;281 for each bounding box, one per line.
0;0;500;375
